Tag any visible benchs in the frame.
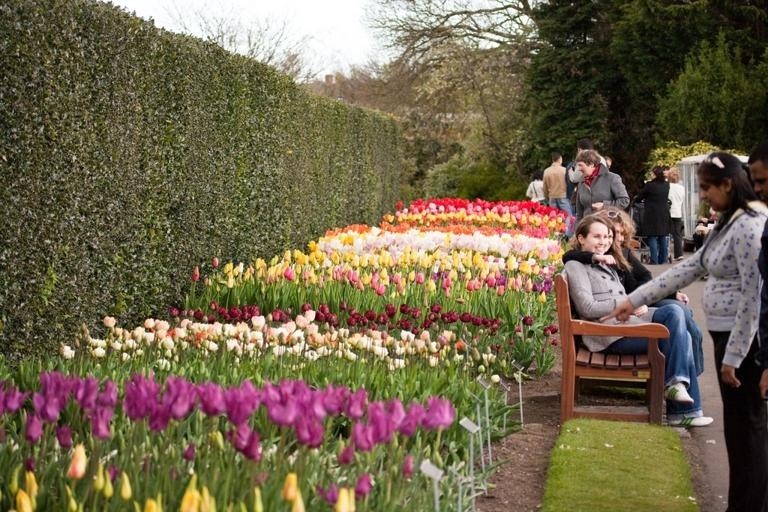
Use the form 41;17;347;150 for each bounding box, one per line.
553;272;671;426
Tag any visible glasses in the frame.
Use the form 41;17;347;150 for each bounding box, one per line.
608;211;623;219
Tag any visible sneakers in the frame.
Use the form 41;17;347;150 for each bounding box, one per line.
669;417;714;427
664;383;695;404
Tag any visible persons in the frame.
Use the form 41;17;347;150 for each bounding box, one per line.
526;139;768;512
